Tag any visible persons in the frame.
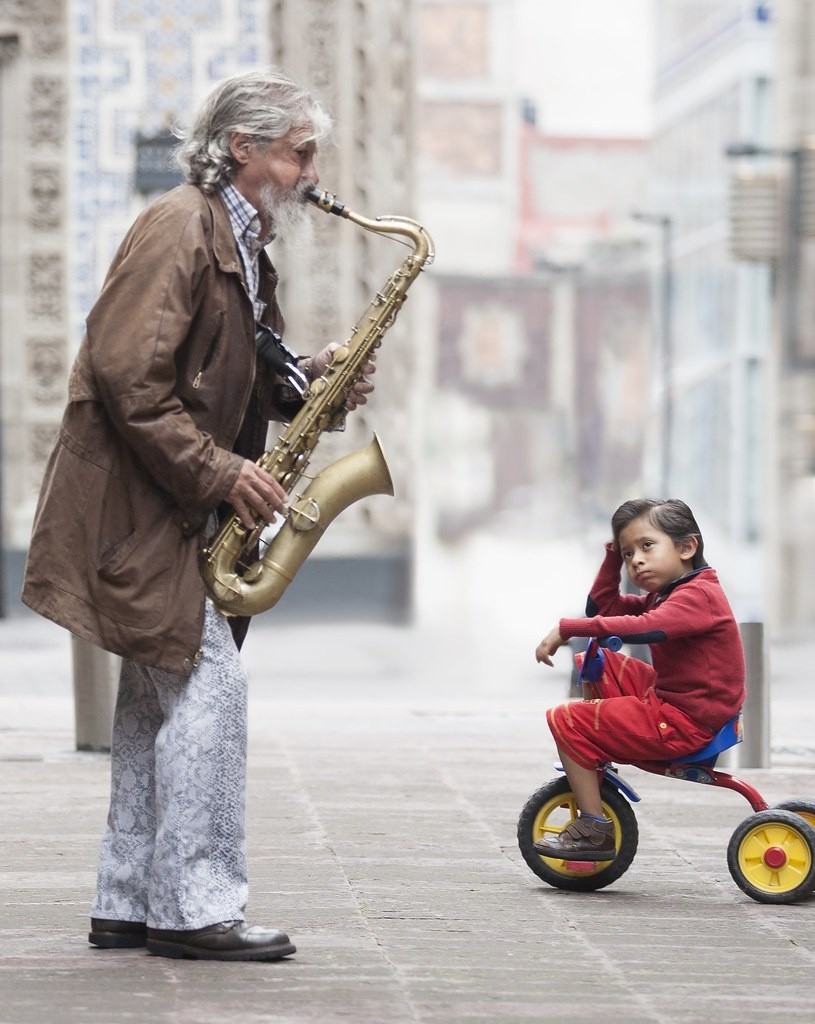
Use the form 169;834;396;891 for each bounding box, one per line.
532;495;745;862
23;70;376;961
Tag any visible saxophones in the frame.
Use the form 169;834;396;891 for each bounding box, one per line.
199;186;435;617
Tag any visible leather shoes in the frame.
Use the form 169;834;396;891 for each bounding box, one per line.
88;918;146;947
146;921;296;959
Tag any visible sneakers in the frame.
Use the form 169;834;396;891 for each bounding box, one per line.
533;816;616;861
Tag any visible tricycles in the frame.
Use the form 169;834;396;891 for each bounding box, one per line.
515;631;815;904
